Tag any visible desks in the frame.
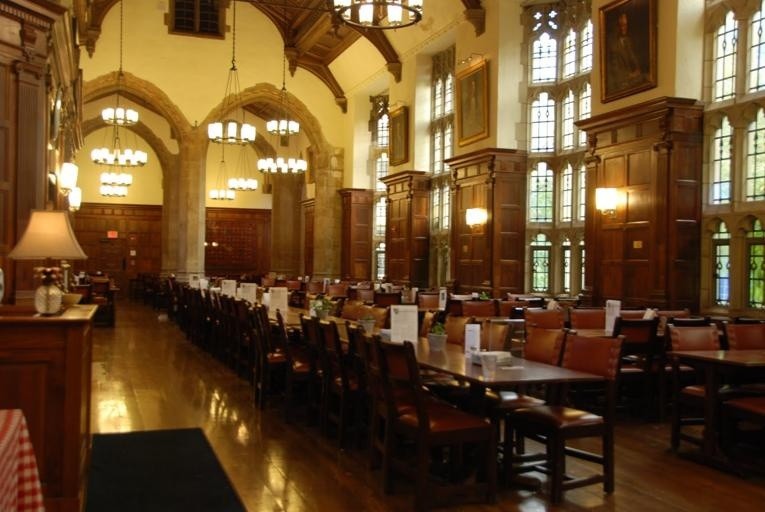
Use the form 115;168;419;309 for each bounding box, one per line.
0;303;100;510
1;410;43;511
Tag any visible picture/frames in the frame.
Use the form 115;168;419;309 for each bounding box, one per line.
388;106;409;166
455;58;490;147
598;1;657;102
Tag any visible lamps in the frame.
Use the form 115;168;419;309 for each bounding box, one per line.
466;208;487;233
49;164;78;198
7;210;87;318
332;0;423;29
67;189;84;215
595;187;616;219
89;1;146;198
208;3;306;200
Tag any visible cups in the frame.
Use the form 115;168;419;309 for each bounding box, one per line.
481;355;497;381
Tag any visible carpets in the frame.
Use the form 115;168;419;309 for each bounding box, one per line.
87;428;247;510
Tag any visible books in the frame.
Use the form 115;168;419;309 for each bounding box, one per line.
472;350;512;365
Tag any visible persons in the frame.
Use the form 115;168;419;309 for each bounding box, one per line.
465;77;478;128
608;11;643;89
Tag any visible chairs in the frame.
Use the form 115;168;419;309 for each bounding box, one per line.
165;270;764;511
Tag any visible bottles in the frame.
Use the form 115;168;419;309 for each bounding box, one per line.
464;345;487;365
372;321;381;336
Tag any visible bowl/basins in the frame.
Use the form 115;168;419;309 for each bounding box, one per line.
63;293;84;307
